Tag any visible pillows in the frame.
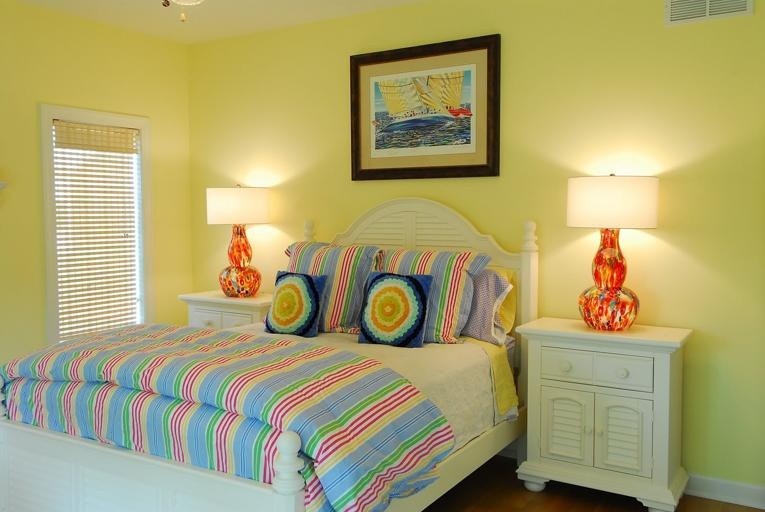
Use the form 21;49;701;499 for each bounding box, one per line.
264;238;518;348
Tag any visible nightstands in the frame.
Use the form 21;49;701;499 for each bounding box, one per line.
178;291;271;330
514;317;692;512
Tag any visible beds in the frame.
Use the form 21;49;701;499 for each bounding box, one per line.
0;197;540;511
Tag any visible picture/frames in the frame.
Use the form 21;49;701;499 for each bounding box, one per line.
348;32;502;183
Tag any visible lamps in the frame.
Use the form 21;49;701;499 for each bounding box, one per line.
565;173;661;332
206;182;269;298
